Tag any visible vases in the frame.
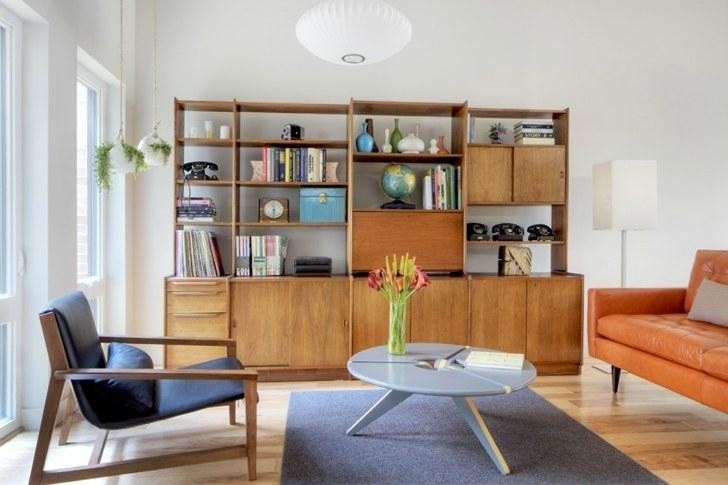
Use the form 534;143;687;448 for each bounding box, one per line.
386;298;409;356
356;114;454;155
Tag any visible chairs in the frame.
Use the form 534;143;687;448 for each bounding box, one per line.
25;287;262;485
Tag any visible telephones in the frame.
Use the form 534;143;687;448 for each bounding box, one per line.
182;161;218;180
527;224;555;241
492;223;524;241
467;223;489;241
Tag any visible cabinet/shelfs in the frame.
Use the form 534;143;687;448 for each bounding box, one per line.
155;270;585;387
165;88;574;282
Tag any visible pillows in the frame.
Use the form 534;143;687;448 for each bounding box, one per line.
683;276;728;329
88;334;163;419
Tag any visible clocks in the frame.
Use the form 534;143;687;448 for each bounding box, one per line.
255;195;290;224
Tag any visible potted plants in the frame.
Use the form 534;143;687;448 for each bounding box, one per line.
135;128;172;171
90;136;151;195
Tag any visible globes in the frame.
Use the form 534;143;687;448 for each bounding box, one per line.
380;163;417;209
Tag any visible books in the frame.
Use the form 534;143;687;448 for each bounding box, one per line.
294;256;332;265
468;113;472;142
471;118;476;142
262;146;326;182
295;264;332;273
295;272;332;277
177;196;287;276
513;119;557;145
466;350;524;370
422;164;461;211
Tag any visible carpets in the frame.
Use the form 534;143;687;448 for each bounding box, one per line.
273;376;670;485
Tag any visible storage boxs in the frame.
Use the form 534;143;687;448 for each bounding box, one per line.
295;184;351;225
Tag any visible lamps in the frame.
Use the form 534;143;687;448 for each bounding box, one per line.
292;0;413;67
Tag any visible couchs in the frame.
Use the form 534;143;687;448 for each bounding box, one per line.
584;248;728;426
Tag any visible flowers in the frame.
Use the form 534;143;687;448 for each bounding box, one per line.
360;246;434;357
